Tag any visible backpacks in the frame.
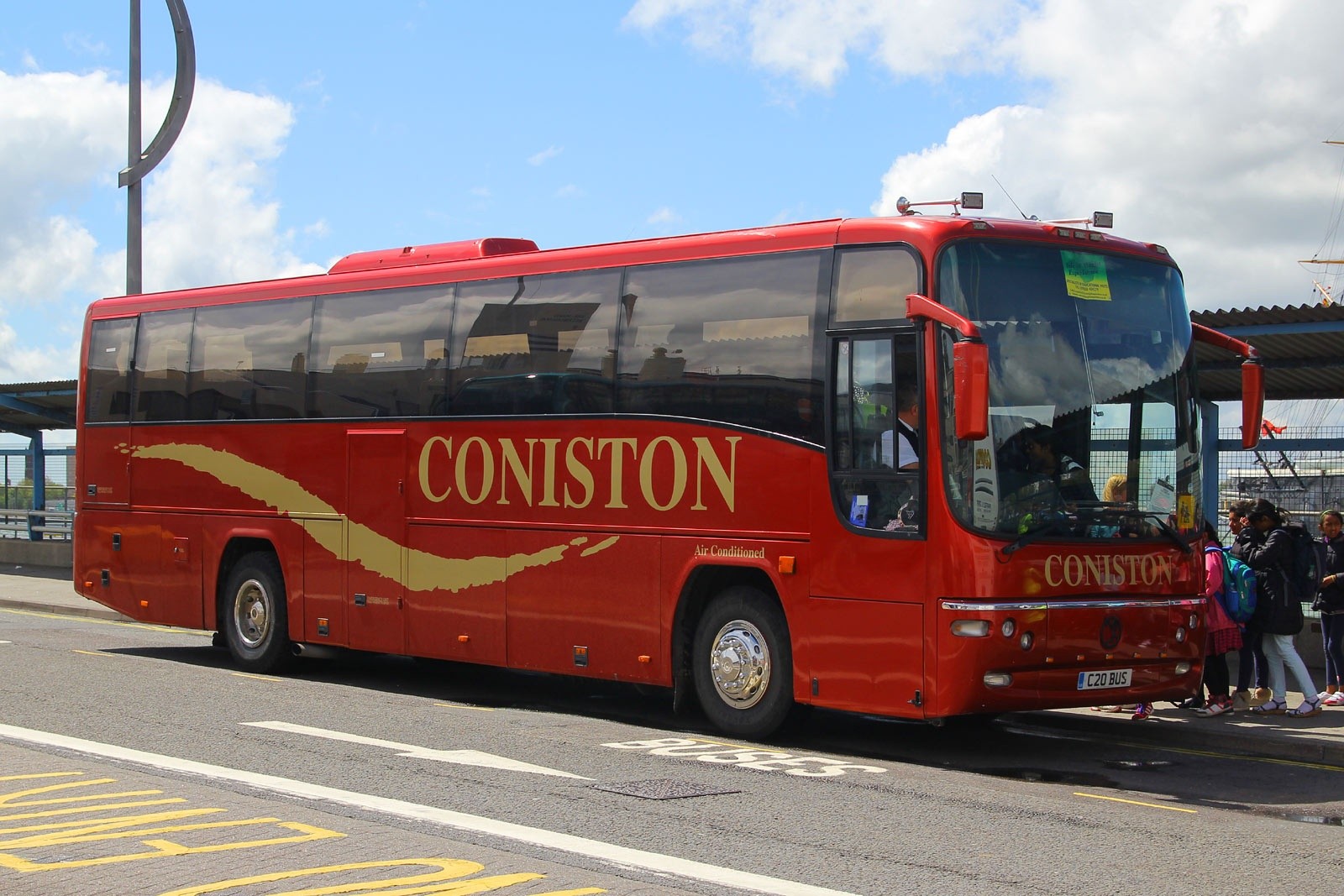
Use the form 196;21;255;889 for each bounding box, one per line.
1269;528;1321;602
1205;546;1256;623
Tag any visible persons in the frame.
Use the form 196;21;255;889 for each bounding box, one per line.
1006;424;1344;720
869;385;923;484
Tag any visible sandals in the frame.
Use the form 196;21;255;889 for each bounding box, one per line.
1289;698;1322;718
1252;700;1286;714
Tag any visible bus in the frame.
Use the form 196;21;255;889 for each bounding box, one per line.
74;191;1265;740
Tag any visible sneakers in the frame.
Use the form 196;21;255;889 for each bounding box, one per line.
1091;706;1122;712
1132;702;1154;720
1317;692;1332;704
1324;692;1344;706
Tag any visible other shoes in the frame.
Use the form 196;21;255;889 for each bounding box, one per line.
1196;694;1233;717
1179;697;1205;708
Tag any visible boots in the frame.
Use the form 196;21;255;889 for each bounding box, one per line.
1250;687;1273;706
1230;690;1250;711
